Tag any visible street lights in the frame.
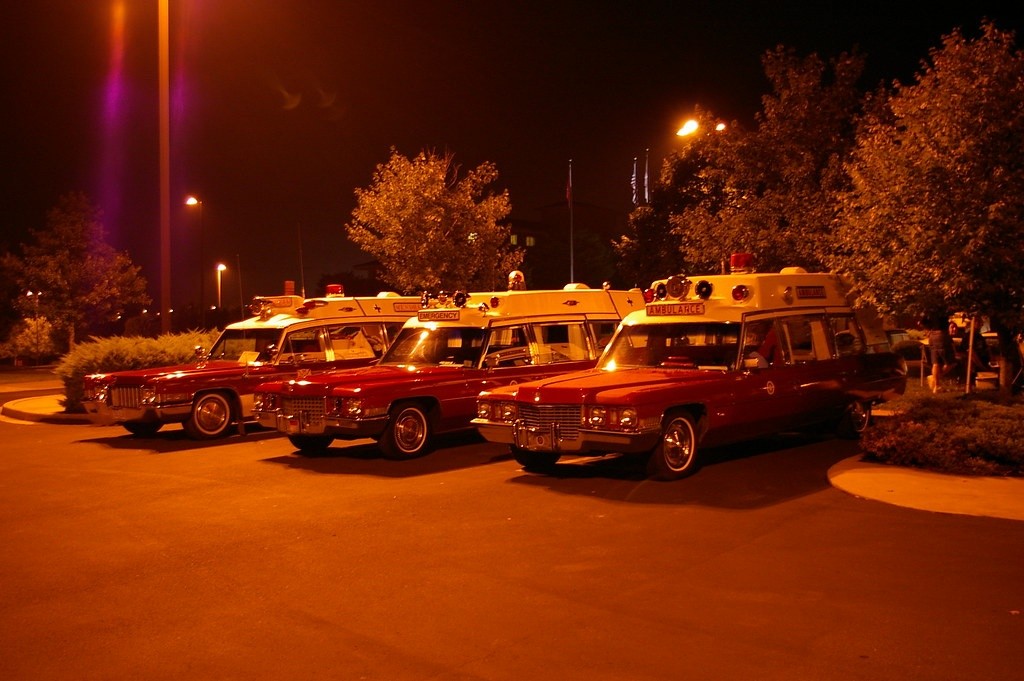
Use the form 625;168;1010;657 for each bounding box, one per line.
217;263;226;310
187;197;205;332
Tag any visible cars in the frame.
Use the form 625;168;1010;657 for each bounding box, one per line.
883;302;1002;362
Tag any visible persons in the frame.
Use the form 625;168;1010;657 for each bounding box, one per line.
917;299;989;391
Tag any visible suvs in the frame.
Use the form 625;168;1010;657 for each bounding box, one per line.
256;271;707;460
469;266;909;485
83;281;520;443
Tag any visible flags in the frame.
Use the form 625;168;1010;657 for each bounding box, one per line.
644;161;648;203
632;163;636;203
566;167;571;206
